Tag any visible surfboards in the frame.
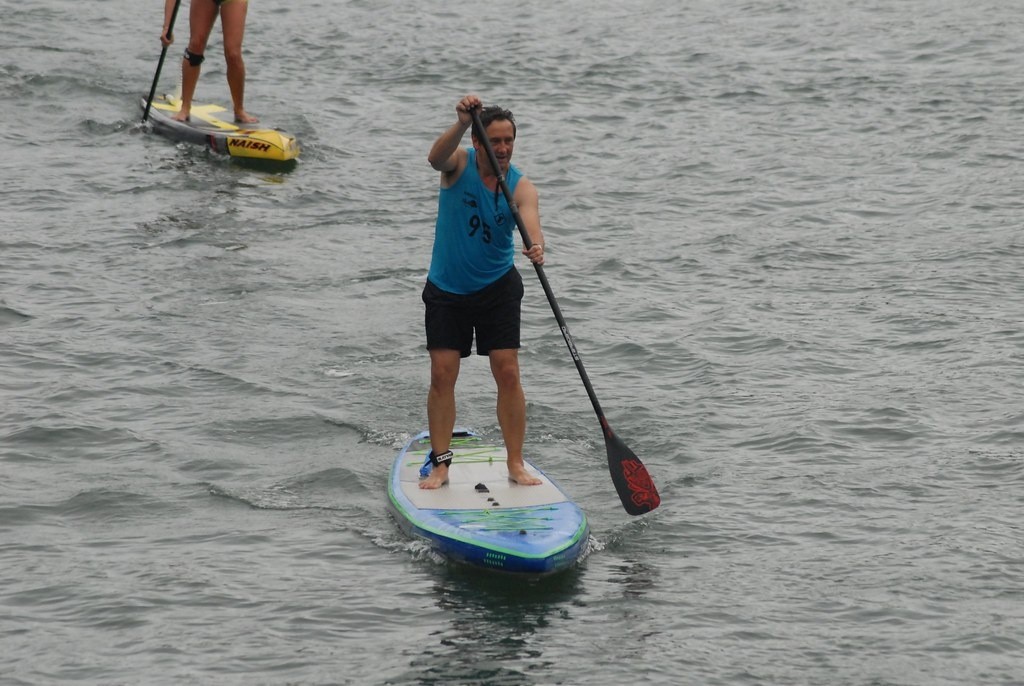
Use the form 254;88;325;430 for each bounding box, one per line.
387;422;592;576
142;88;301;165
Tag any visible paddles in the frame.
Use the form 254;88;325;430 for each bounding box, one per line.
141;2;184;126
467;103;662;517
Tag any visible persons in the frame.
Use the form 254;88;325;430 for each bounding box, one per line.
160;0;259;123
418;96;544;490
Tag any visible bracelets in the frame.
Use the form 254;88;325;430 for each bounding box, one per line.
533;244;544;255
